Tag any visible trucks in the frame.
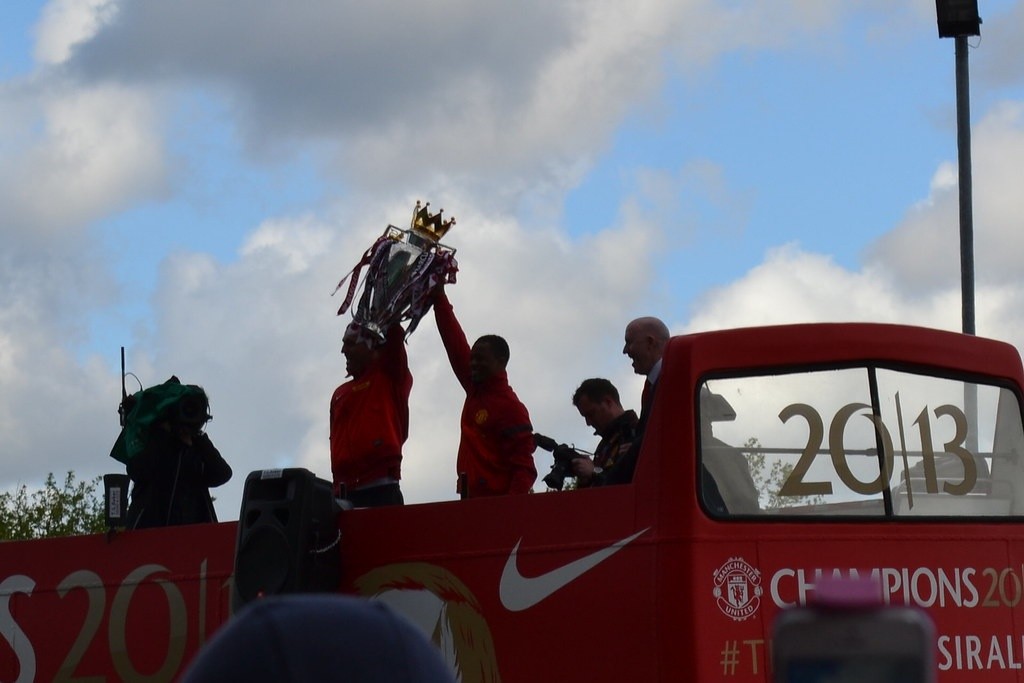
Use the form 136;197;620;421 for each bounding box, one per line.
0;325;1024;683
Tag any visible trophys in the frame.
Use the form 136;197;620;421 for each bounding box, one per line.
332;200;460;344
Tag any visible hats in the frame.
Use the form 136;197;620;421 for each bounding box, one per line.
183;386;211;423
183;594;457;683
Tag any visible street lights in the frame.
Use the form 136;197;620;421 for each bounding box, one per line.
935;1;982;332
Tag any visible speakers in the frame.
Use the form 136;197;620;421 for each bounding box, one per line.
231;468;340;617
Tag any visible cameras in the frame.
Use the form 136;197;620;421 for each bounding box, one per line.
534;432;586;491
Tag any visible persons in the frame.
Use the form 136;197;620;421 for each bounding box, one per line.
119;386;231;531
434;284;538;501
330;318;412;508
571;378;639;487
623;317;713;484
180;594;457;683
